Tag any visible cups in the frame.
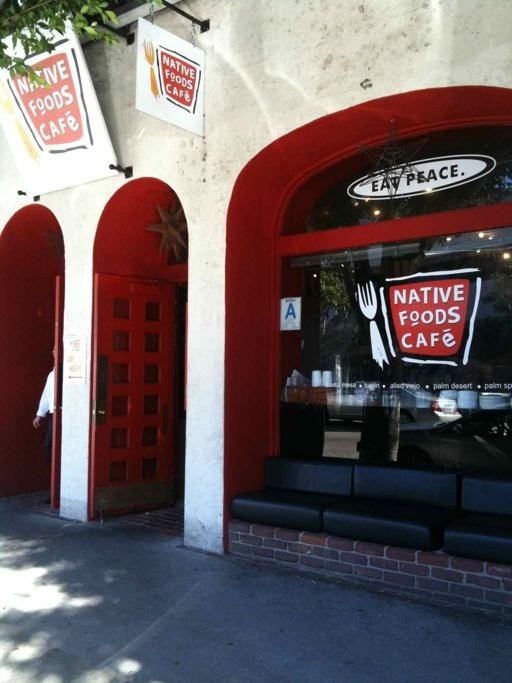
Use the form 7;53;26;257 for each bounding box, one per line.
309;371;334;388
457;391;479;411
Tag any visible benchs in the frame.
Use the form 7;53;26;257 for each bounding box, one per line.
232;456;512;561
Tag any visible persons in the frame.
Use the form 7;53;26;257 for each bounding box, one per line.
33;346;55;504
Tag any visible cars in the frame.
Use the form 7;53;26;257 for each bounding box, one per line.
327;380;463;424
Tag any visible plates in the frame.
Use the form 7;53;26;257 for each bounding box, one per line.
478;392;509;413
